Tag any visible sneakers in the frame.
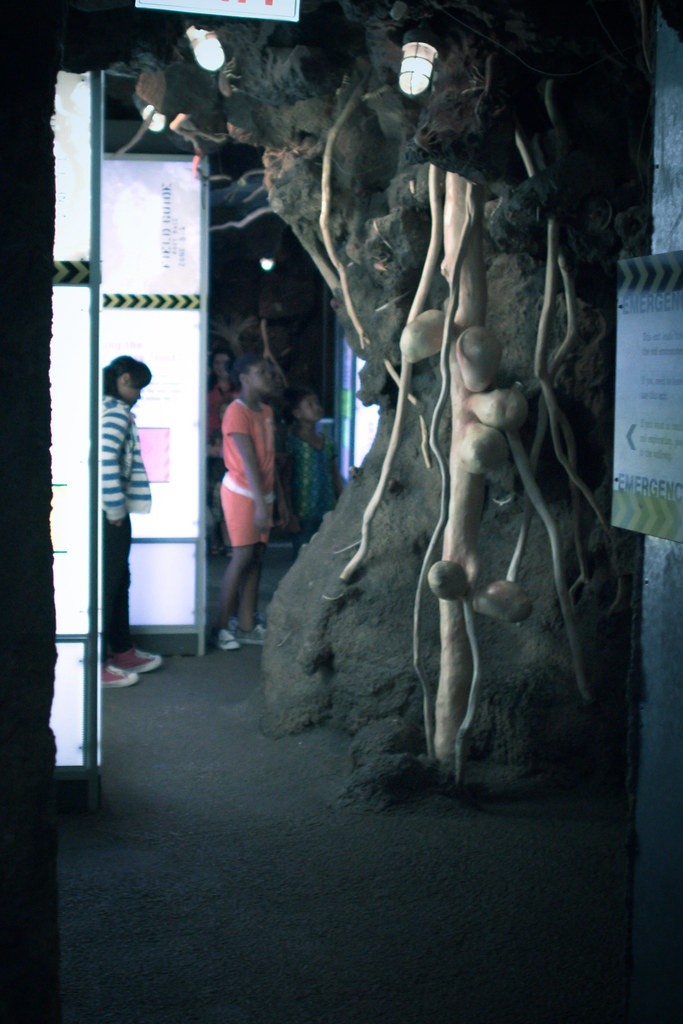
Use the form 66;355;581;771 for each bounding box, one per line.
214;627;240;651
234;623;266;647
102;660;140;688
111;646;164;674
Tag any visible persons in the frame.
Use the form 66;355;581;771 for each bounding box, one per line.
101;356;161;687
209;318;341;647
